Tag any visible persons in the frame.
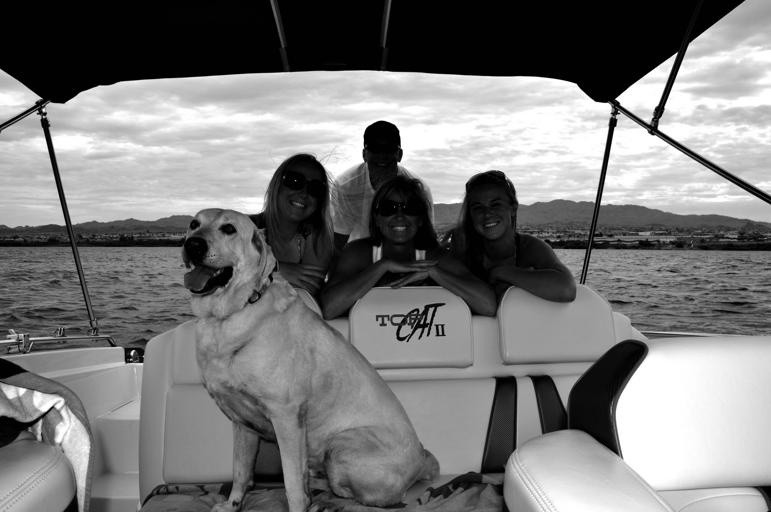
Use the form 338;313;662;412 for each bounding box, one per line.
327;119;438;280
438;167;578;303
312;172;498;322
247;152;335;301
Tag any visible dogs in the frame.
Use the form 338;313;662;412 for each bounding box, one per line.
181;208;440;512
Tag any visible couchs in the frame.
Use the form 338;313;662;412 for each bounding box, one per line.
134;282;654;511
504;334;770;511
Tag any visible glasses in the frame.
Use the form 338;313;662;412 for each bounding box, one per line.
380;198;424;217
364;141;399;154
282;171;326;198
465;170;505;198
